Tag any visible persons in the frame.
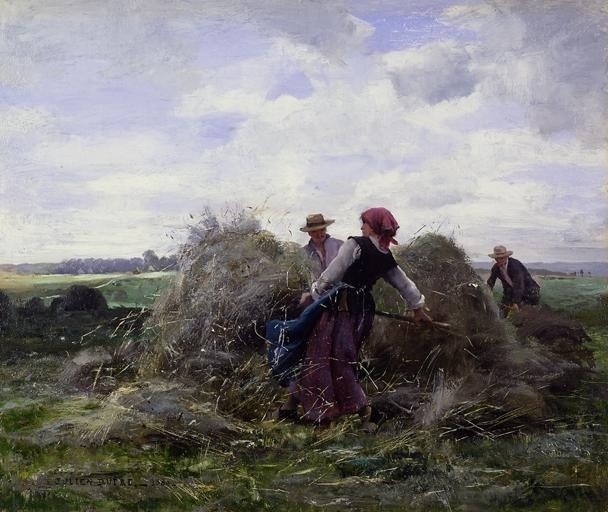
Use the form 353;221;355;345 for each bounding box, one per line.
265;206;432;435
298;214;345;277
486;246;540;317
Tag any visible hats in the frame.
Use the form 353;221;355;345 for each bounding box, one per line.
488;245;512;258
300;214;335;232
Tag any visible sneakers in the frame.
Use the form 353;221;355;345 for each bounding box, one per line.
359;406;381;433
272;404;300;421
